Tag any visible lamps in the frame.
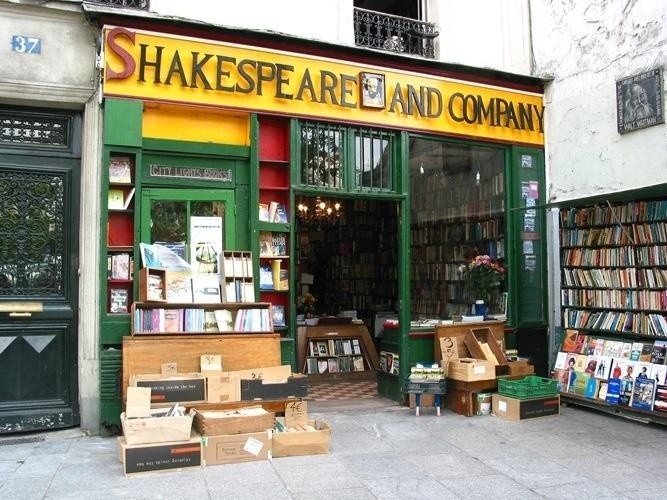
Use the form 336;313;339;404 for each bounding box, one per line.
299;195;342;232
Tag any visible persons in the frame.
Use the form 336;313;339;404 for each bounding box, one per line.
623;84;653;123
364;78;382;106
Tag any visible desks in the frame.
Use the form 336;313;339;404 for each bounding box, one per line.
433;320;535;417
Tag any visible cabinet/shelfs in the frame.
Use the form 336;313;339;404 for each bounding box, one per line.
99;143;142;342
551;178;667;429
129;251;276;334
322;165;506;319
257;158;291;332
296;323;378;382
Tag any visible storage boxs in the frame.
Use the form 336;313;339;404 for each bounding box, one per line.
464;326;509;375
492;392;561;420
116;371;332;477
441;357;497;381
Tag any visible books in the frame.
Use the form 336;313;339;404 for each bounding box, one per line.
118;402;332;474
109;188;123;208
295;150;510;394
122;187;135;207
110;157;131;183
552;202;667;410
108;200;289;333
126;386;150;417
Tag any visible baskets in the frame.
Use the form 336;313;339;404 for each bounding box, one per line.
402;373;446;395
498;376;559;400
